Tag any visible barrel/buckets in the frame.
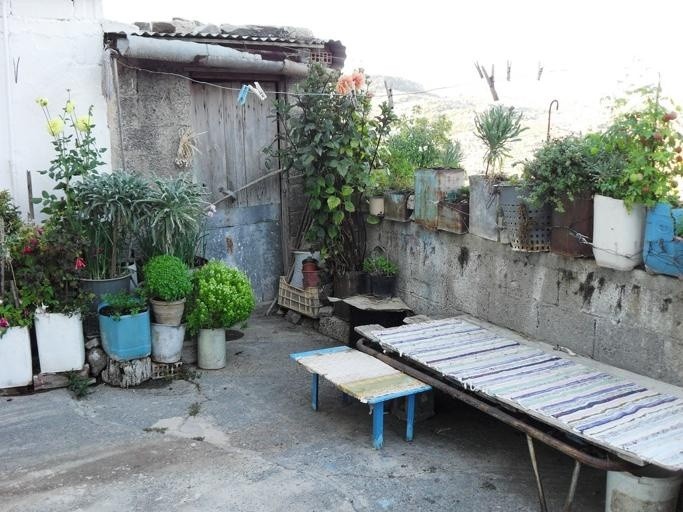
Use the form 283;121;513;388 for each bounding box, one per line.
78;267;131;337
34;307;85;372
97;298;151;361
605;470;683;512
413;166;683;279
0;325;33;389
198;328;226;369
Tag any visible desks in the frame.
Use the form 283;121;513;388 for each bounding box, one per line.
285;348;431;447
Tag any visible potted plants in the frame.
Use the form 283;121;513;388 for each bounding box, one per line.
0;85;135;391
95;290;154;365
184;257;256;372
414;84;681;282
140;253;194;326
264;67;417;323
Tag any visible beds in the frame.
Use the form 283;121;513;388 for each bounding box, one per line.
343;315;683;512
